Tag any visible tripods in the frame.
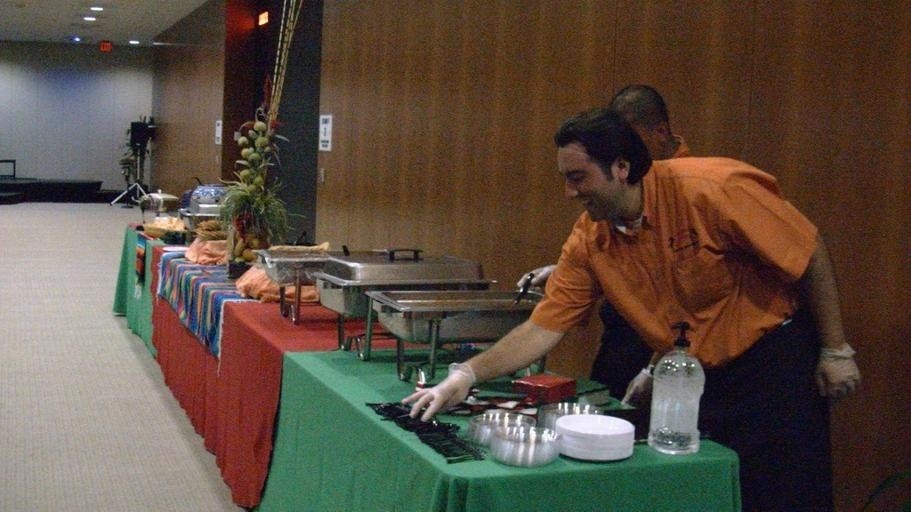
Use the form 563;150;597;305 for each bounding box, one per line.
110;150;147;206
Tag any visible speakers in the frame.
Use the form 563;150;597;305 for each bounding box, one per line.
131;122;149;149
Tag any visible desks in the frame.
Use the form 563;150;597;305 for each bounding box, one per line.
127;223;742;512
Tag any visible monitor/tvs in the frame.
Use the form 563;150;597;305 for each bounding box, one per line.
0;160;15;180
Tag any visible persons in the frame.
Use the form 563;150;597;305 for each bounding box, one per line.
516;84;727;449
400;107;863;511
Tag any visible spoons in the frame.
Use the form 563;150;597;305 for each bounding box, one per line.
366;396;487;463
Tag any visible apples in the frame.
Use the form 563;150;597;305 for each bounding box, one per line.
238;121;270;193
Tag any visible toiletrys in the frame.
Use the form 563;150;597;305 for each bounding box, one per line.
647;321;706;456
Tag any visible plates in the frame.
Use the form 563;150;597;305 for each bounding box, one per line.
466;402;636;469
